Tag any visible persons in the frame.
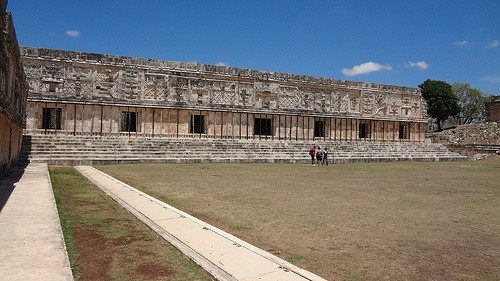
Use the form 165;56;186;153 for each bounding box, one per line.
309;146;316;166
322;147;329;166
316;146;323;166
